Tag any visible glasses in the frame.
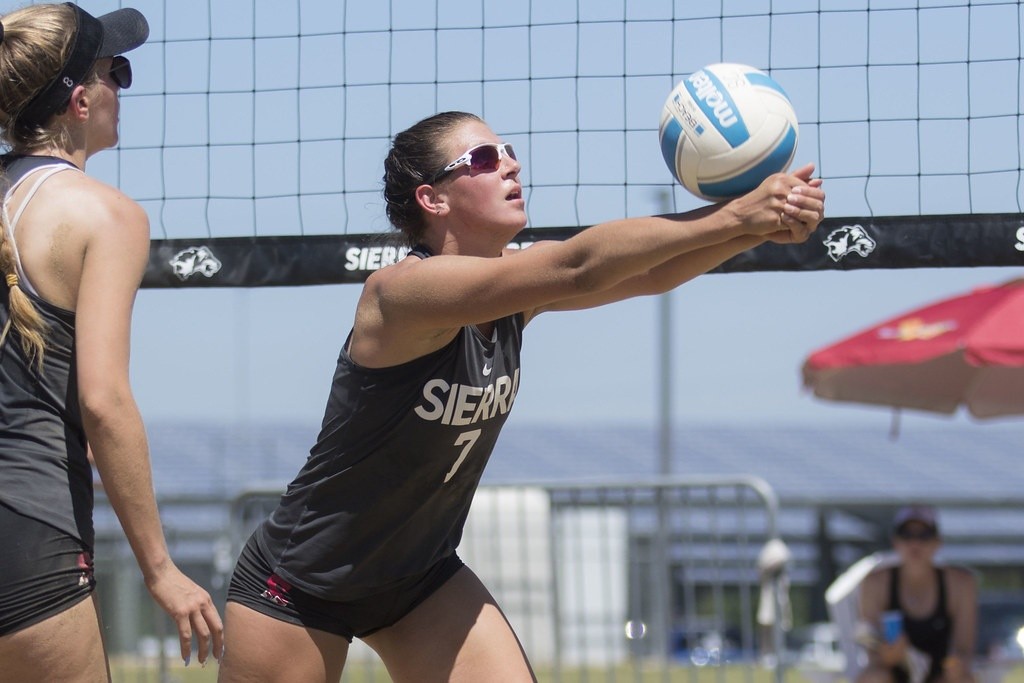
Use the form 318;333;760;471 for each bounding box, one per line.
897;528;933;541
404;143;517;205
56;55;132;116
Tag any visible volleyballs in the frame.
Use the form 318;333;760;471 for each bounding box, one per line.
657;62;801;204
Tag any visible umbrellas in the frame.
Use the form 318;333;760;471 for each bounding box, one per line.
804;276;1024;422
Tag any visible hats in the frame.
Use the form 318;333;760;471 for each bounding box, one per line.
17;2;150;124
891;504;934;532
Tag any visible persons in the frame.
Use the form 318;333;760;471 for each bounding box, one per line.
216;111;818;683
841;507;979;683
0;1;224;681
